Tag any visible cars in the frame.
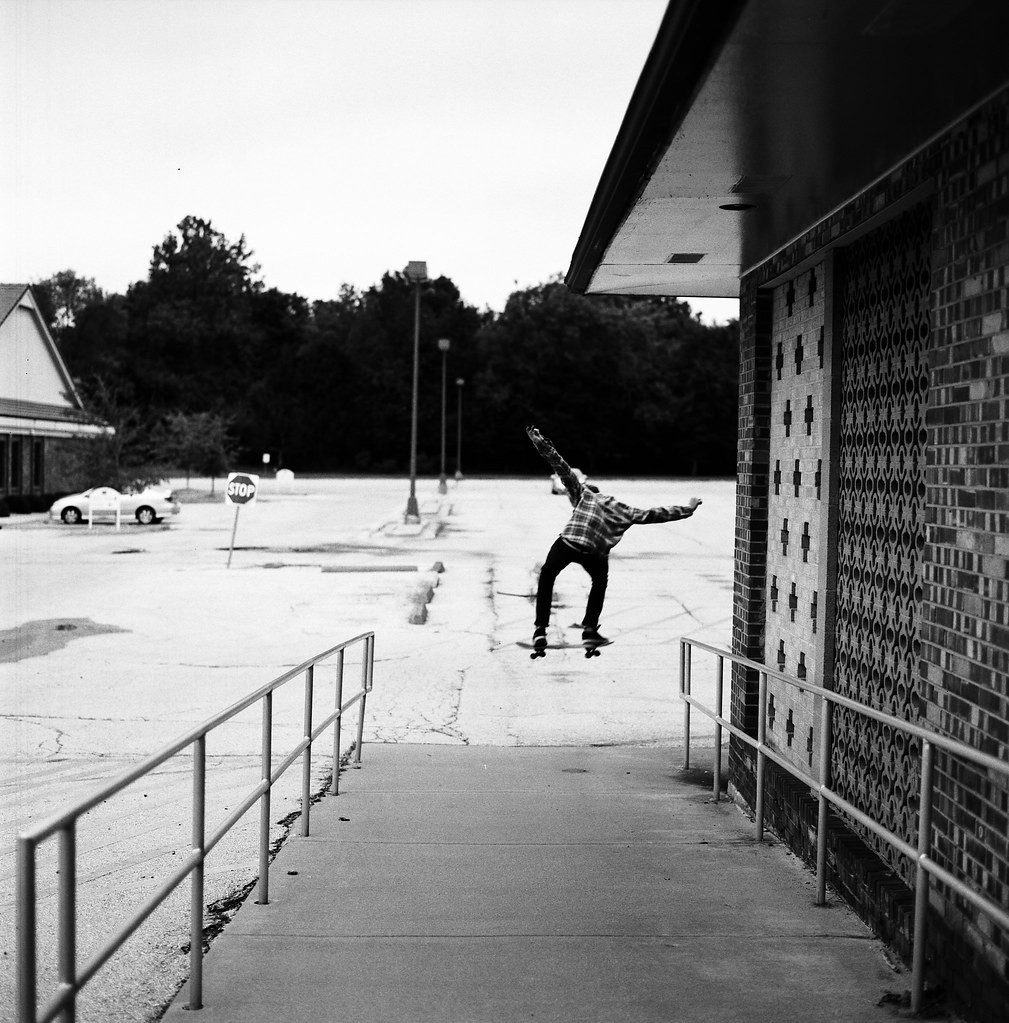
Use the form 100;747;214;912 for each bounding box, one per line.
49;479;181;526
550;468;588;495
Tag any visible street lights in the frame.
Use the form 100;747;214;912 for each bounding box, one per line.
438;339;450;494
454;378;464;479
405;262;428;523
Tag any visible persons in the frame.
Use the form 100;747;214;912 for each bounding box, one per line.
526;426;703;647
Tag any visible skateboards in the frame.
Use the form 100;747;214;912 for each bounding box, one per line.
515;640;615;659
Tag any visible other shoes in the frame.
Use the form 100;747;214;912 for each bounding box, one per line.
533;628;547;648
582;624;608;644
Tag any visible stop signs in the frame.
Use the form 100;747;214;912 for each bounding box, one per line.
226;472;260;508
